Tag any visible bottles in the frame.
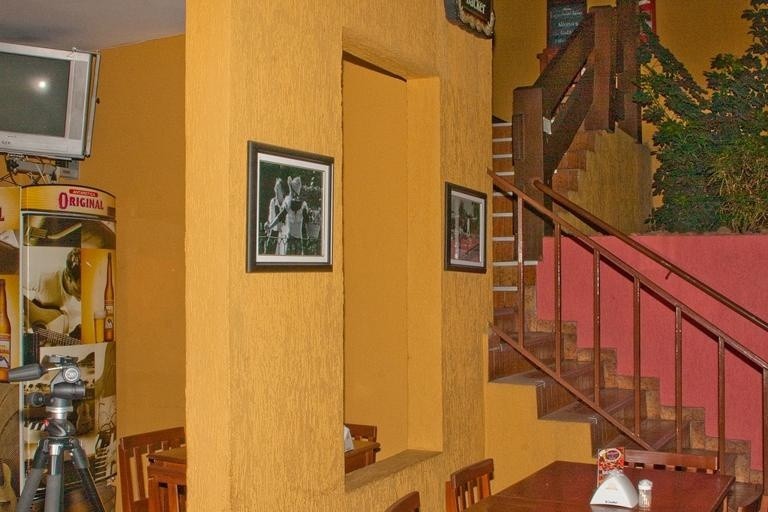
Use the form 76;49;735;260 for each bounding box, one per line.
287;176;301;195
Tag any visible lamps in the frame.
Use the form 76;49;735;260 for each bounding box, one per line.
737;492;763;512
345;422;378;464
119;426;184;511
386;491;419;511
446;459;494;512
625;450;718;473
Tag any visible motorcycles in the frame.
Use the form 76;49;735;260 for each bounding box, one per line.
16;436;105;512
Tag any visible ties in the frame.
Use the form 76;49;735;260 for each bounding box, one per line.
0;40;101;161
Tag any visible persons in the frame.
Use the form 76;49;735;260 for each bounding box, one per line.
264;175;310;255
263;178;287;254
25;247;81;348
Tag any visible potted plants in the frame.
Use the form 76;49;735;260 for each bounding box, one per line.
245;140;334;272
443;181;486;274
546;0;587;48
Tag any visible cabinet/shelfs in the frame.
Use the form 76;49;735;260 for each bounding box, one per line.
637;479;653;507
103;252;114;342
